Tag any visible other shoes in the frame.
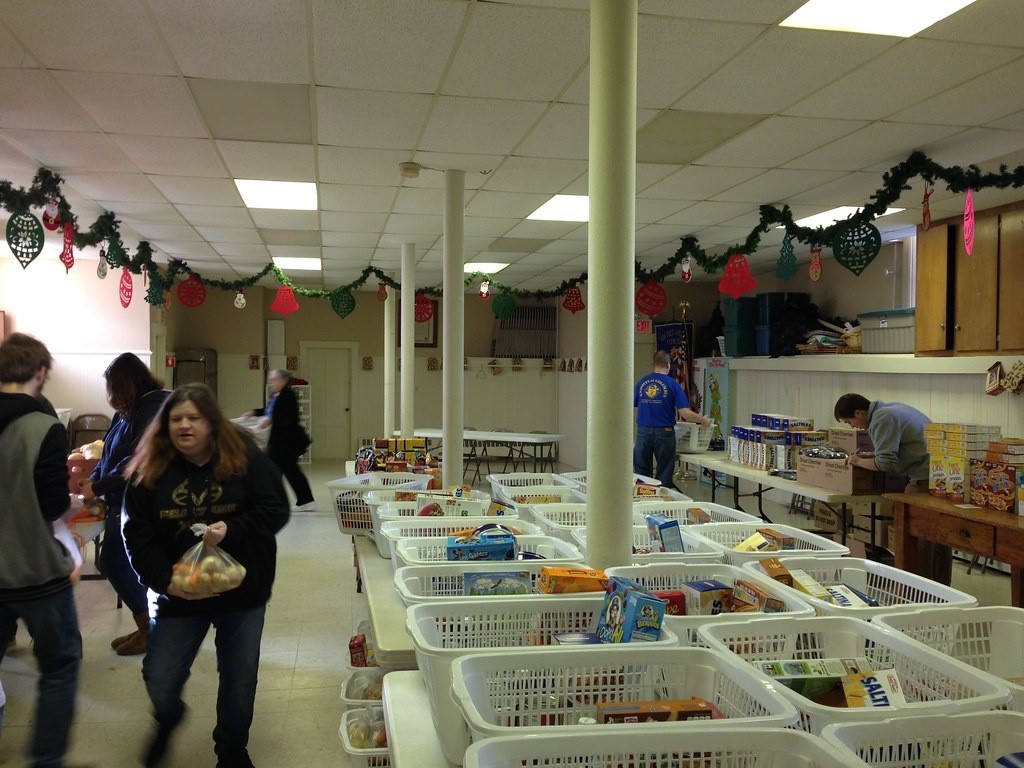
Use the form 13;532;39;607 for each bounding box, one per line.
143;700;185;766
215;750;254;768
288;502;314;512
7;627;17;648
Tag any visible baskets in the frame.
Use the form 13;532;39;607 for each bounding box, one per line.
319;456;1024;767
674;416;713;452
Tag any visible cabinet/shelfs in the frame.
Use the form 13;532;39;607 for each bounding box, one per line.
914;199;1024;358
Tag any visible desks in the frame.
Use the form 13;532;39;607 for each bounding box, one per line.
345;460;412;497
64;493;123;609
390;428;568;486
882;492;1024;609
700;460;883;558
382;671;464;768
353;500;581;669
675;450;728;503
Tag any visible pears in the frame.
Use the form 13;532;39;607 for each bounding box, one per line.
90;497;105;516
172;554;245;593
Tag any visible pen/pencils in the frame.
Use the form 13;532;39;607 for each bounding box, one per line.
854;448;861;454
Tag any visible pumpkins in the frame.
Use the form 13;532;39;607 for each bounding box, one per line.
68;440;104;472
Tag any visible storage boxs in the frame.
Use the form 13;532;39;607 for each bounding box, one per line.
66;458;102;494
856;307;915;353
722;291;811;356
291;385;312;464
338;459;908;767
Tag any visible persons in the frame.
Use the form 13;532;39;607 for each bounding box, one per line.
834;393;952;588
121;382;291;768
0;333;84;768
634;351;711;488
242;369;317;511
79;353;173;655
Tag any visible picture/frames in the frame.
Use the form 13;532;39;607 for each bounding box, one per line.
397;298;438;348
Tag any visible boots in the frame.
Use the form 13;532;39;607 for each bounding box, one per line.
110;611;150;655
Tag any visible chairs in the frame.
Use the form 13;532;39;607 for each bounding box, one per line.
64;417;73;452
515;430;554;473
472;428;516;487
72;414;111;449
435;427;481;482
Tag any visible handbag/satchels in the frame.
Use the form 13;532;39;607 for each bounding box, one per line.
170;524;244;595
296;425;312;454
